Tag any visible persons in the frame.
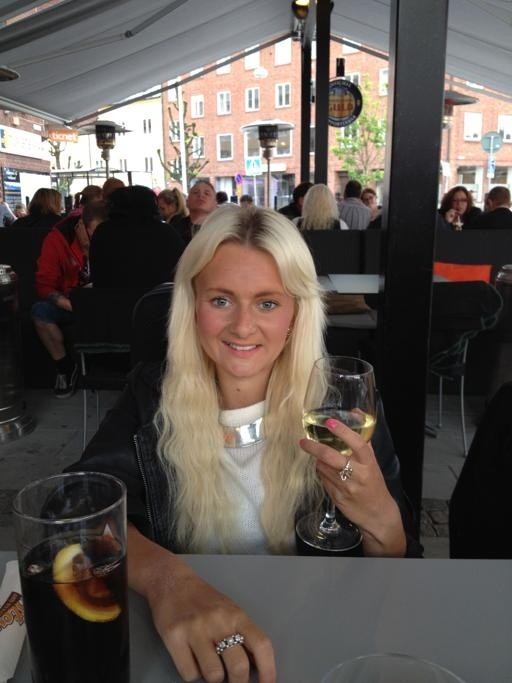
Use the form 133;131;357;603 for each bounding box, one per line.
273;178;391;366
1;176;256;397
41;203;424;682
434;184;511;273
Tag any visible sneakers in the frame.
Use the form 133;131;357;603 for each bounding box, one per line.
54;363;80;399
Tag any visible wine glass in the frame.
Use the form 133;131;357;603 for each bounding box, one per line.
300;355;382;550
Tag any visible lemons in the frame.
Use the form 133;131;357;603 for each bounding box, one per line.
52;543;122;623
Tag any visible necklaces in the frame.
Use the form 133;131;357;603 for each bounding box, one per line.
210;408;267;451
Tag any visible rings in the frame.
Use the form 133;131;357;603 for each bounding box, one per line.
214;631;245;653
338;455;354;482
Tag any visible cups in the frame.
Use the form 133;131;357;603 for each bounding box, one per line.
453;222;464;232
11;471;126;679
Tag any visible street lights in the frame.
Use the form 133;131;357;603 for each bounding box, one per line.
80;119;129;177
238;115;295;204
